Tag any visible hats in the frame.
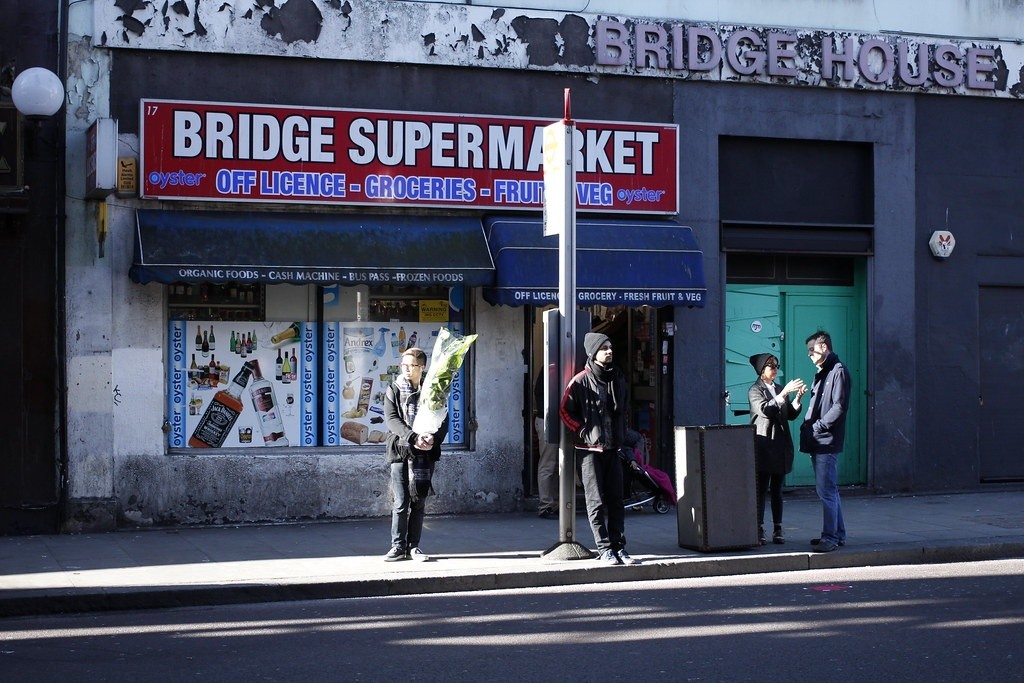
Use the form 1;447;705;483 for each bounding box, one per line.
584;333;612;360
750;353;773;375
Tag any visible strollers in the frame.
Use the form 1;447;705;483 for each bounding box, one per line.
616;447;670;514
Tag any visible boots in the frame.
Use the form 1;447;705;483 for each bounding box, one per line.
758;527;767;545
773;525;784;544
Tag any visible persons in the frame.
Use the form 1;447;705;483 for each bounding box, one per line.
382;348;449;562
535;332;635;565
748;353;808;546
799;330;851;553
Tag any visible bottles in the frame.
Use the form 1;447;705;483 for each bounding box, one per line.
387;366;394;375
399;327;406;352
394;366;399;375
407;332;417;349
391;333;400;358
188;322;301;447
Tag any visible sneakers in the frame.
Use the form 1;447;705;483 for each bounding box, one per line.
600;549;635;564
540;507;559;520
383;546;429;561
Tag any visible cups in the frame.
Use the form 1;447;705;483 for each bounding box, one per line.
238;426;253;443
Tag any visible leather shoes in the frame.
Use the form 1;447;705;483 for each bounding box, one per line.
811;538;846;546
813;540;839;553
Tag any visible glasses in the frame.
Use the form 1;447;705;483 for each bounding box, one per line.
765;364;780;369
401;363;422;369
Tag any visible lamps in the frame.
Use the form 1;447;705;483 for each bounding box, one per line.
10;65;64;146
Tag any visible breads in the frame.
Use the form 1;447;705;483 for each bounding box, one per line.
368;430;387;443
340;422;368;445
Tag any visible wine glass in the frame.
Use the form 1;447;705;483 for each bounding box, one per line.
195;396;202;415
286;394;295;416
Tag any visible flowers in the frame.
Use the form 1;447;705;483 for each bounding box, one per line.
418;330;482;409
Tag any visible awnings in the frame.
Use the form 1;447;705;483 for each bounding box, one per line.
482;214;706;310
128;208;496;287
